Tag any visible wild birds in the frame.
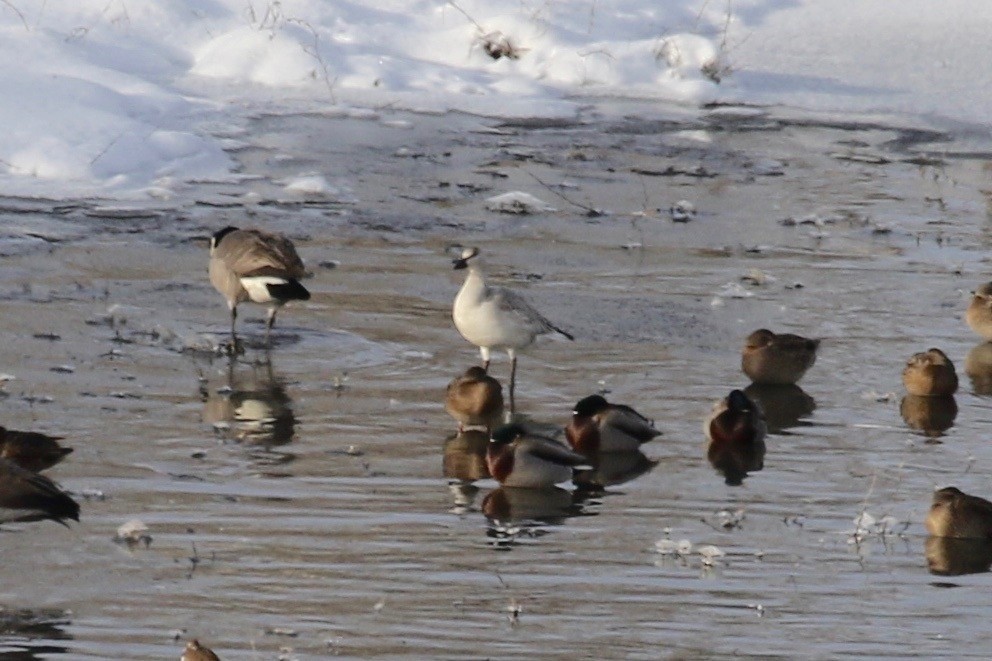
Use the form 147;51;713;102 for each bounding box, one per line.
925;486;992;540
0;426;74;472
452;245;573;413
208;225;311;346
445;282;991;488
